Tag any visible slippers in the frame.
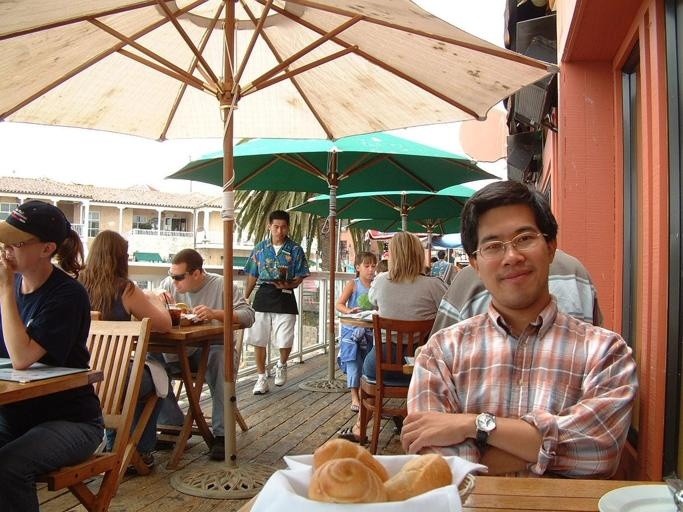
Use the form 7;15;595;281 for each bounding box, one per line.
336;426;371;444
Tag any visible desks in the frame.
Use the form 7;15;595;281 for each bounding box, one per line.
0;364;105;406
338;312;374;329
232;472;683;512
134;319;252;472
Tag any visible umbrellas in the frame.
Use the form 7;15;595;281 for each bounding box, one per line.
342;216;462;249
288;184;480;230
0;0;562;499
165;132;500;394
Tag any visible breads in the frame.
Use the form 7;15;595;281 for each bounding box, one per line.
174;303;189;312
313;438;390;482
381;453;452;500
309;458;389;503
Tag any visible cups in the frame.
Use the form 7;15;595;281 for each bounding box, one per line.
277;265;288;279
166;303;182;327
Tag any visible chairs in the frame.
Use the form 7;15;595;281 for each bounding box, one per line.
37;306;161;512
355;312;438;454
88;309;154;477
169;296;253;435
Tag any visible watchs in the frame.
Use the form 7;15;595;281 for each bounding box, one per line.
474;410;496;456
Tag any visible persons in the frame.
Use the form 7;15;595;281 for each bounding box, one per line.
399;181;638;480
76;230;172;475
133;248;255;461
336;230;448;442
429;248;603;339
376;249;461;285
243;210;310;394
0;201;103;510
335;250;377;413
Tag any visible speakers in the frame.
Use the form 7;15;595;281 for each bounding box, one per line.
513;35;557;130
507;144;535;184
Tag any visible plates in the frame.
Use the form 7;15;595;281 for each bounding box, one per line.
596;484;682;511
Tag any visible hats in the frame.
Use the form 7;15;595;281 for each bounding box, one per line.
1;200;69;246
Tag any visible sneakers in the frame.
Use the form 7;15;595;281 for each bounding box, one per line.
127;453;155;471
211;435;224;459
253;376;269;395
274;362;287;386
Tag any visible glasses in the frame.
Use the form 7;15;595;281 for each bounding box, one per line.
167;267;196;282
471;230;551;263
